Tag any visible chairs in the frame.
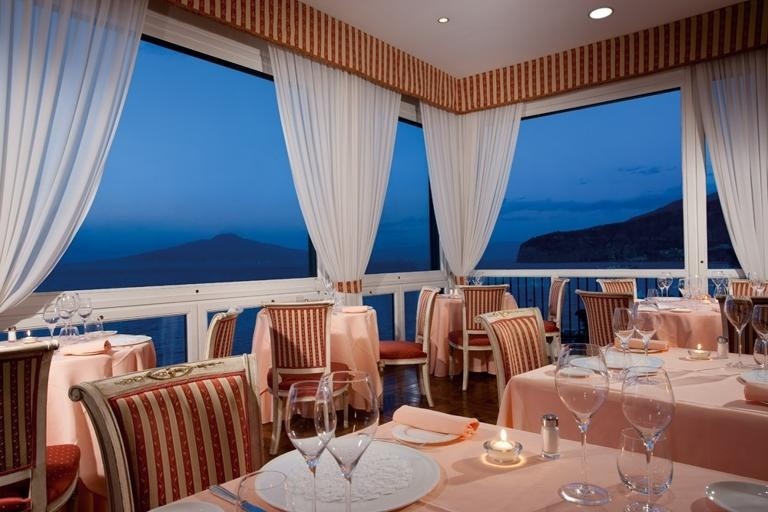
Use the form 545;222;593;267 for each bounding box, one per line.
1;344;81;510
69;353;264;511
203;302;382;455
377;277;768;408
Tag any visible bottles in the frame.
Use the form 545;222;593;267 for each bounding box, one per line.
6;326;18;343
715;335;729;358
537;413;561;460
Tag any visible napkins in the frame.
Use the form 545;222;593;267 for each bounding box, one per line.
60;340;111;356
392;405;480;438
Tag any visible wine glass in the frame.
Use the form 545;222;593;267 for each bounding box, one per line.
723;296;768;372
614;303;659;368
646;271;768;302
551;343;678;512
284;370;379;510
40;294;92;343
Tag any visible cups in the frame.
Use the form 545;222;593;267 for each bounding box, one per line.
483;441;523;464
688;350;712;361
236;469;288;512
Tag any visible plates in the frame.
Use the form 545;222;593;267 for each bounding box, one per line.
88;329;152;346
671;308;692;314
254;438;443;512
705;479;767;511
389;421;470;444
339;306;367;313
740;369;768;384
145;501;224;512
